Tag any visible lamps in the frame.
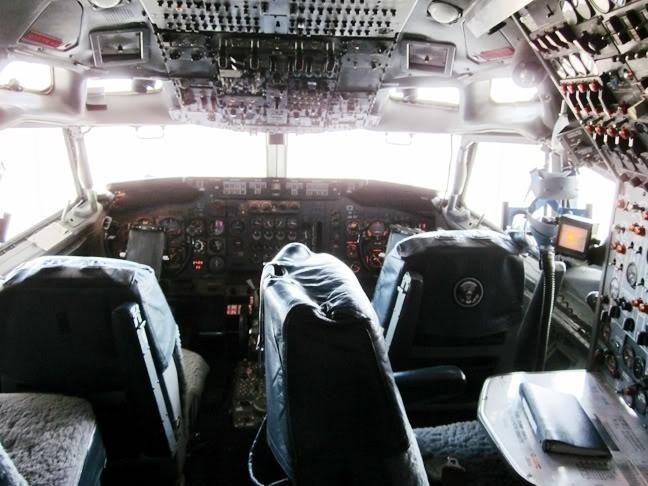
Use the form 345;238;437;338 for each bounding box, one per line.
510;37;553;89
427;1;463;25
86;0;132;12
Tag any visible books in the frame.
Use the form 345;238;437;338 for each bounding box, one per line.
519;382;611;459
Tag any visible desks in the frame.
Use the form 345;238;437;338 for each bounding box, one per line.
477;367;648;486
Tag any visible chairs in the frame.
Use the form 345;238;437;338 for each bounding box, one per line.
248;239;533;483
1;252;207;484
370;229;534;431
